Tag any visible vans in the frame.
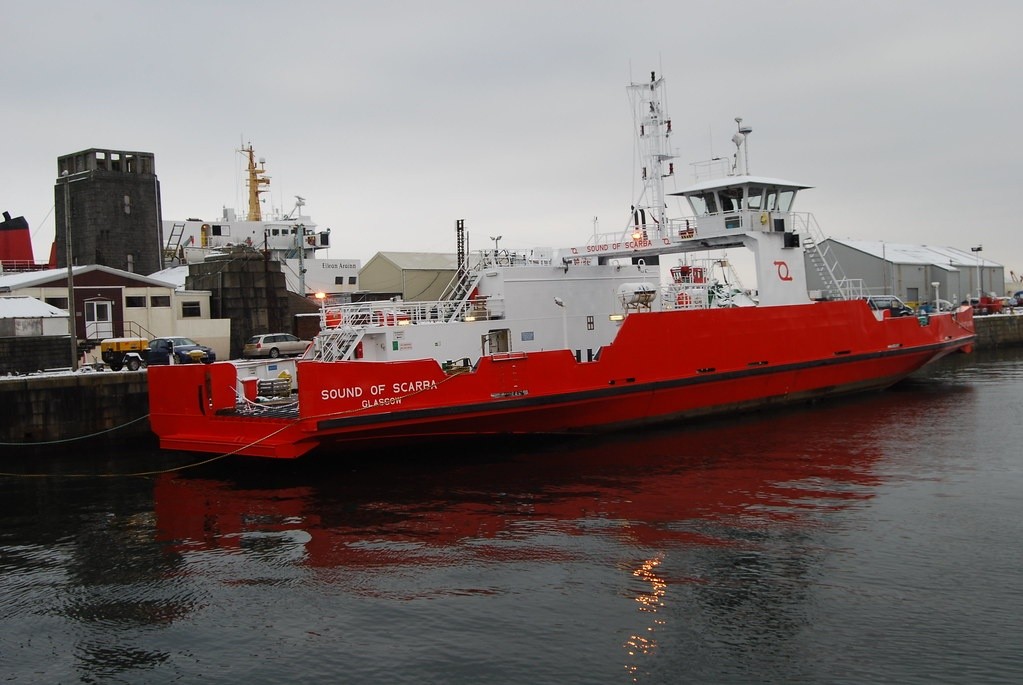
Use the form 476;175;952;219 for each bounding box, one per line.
862;297;914;318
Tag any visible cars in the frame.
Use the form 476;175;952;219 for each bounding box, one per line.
145;338;216;365
243;333;313;357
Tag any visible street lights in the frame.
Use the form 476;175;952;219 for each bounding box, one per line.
971;247;982;301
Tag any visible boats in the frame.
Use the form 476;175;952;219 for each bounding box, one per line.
146;65;981;460
